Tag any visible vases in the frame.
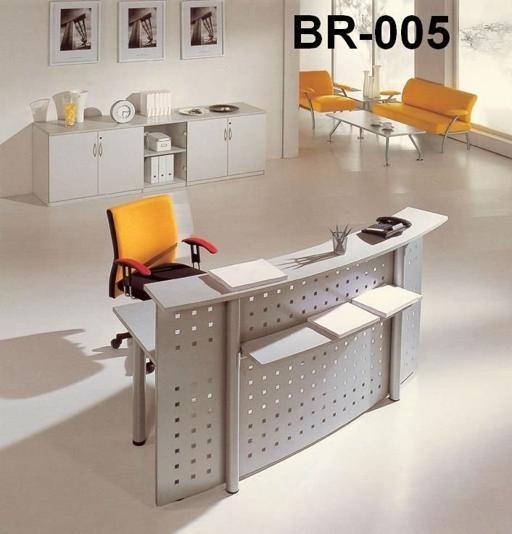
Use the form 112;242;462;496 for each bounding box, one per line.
363;63;381;98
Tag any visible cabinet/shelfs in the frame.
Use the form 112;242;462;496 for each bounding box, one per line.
33;102;268;206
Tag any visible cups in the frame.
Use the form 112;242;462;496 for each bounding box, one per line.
63;90;88;129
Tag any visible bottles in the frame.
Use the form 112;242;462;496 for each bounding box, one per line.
363;65;381;98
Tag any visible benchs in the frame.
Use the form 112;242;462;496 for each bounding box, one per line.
371;76;478;154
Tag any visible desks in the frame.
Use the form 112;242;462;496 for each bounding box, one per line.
111;206;450;506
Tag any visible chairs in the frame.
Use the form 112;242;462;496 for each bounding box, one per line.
102;191;221;373
297;69;362;131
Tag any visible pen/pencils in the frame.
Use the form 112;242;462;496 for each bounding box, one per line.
328;223;352;252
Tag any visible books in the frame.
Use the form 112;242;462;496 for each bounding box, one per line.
207;259;288;292
307;285;421;339
140;89;171;117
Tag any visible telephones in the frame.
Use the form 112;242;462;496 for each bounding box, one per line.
362;217;412;239
